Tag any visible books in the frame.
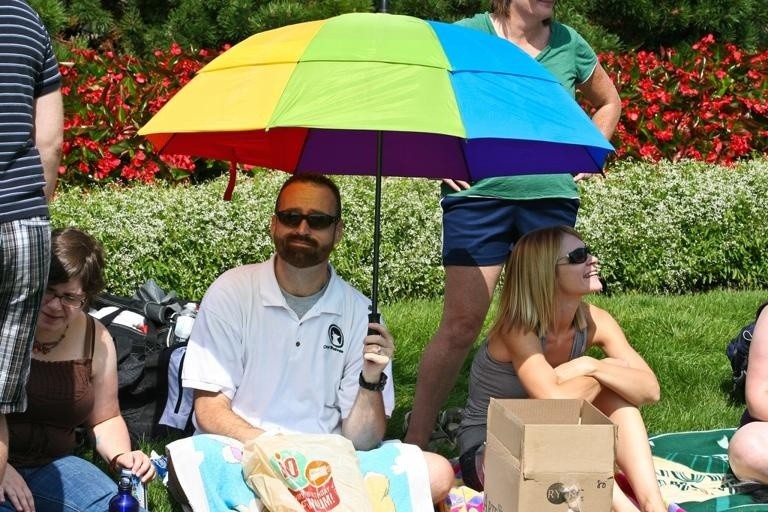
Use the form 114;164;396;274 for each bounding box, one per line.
483;397;616;512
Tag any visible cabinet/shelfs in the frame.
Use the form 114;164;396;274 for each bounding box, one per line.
108;474;139;512
169;300;200;347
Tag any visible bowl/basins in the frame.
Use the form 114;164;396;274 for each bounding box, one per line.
33;323;68;354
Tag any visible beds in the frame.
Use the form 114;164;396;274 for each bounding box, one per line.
137;0;616;345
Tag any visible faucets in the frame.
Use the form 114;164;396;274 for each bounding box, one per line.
399;406;471;464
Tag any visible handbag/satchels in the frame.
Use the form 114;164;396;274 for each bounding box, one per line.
108;454;125;476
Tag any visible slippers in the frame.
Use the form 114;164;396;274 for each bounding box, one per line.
724;320;750;402
91;275;200;437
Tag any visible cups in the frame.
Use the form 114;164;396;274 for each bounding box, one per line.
555;244;594;268
42;287;87;308
274;207;341;232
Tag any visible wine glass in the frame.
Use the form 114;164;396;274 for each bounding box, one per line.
377;346;381;355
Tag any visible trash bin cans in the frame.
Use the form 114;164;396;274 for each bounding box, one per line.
359;370;387;391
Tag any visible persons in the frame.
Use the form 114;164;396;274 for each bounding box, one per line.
728;302;768;486
170;174;455;512
404;0;623;452
0;228;159;512
458;225;668;512
0;1;65;490
